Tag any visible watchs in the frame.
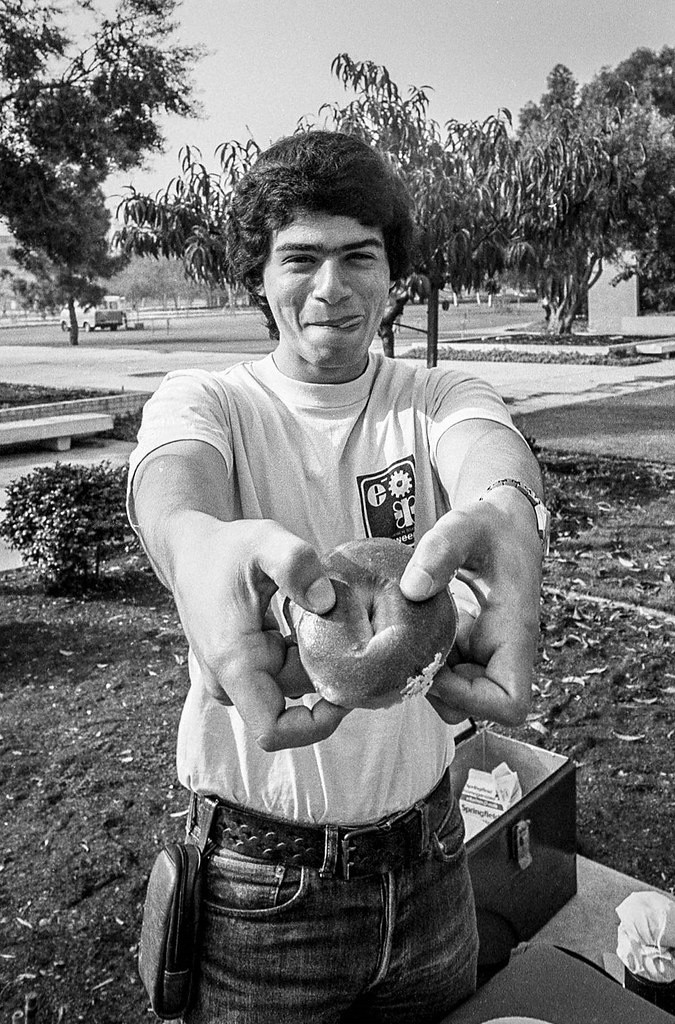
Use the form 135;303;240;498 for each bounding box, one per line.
478;478;550;558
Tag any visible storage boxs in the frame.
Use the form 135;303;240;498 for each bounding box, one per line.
446;717;578;995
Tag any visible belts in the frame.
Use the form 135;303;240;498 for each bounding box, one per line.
186;768;451;880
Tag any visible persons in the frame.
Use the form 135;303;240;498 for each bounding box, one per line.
124;132;544;1024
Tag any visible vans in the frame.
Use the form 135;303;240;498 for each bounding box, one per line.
59;295;124;332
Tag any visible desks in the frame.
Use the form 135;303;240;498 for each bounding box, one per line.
440;943;675;1024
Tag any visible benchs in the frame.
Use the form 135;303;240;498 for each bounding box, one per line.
636;340;675;359
527;853;675;986
0;412;114;451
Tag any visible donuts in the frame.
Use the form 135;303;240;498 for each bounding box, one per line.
287;538;458;712
